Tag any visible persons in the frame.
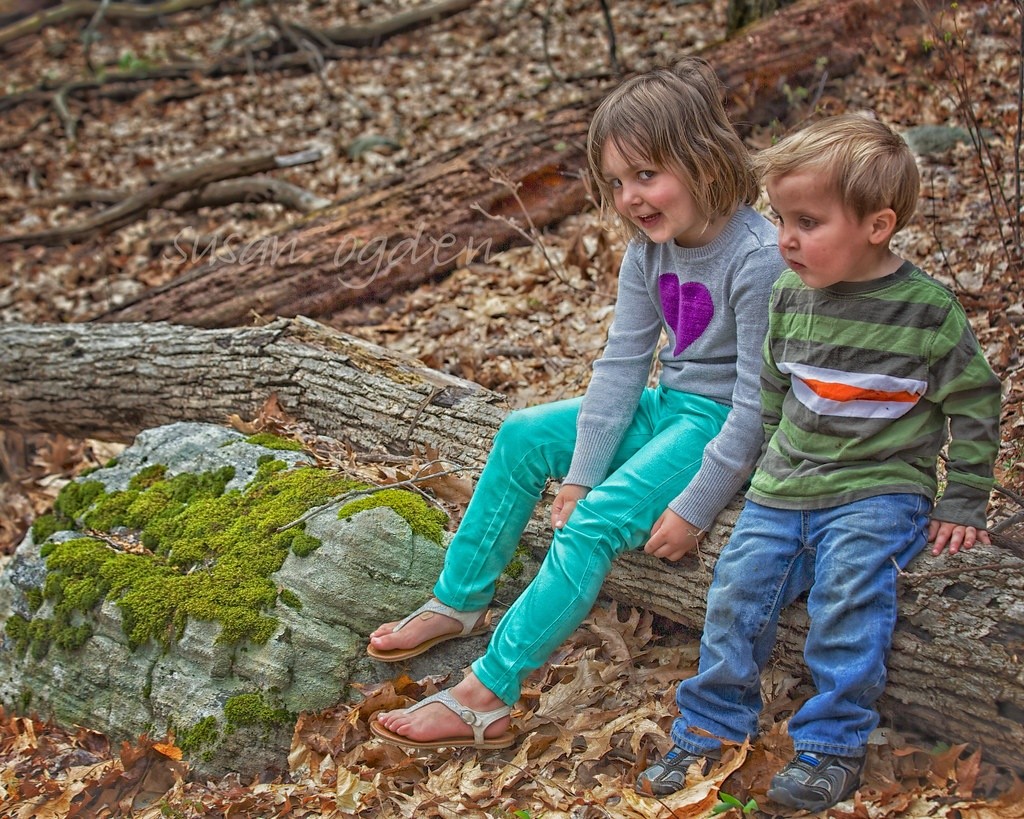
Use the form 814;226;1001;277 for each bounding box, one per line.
636;111;1002;809
368;56;797;750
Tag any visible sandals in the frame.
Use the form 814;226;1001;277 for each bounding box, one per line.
369;689;515;749
367;603;493;663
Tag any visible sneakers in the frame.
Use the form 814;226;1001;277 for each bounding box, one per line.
634;744;714;795
765;747;868;810
